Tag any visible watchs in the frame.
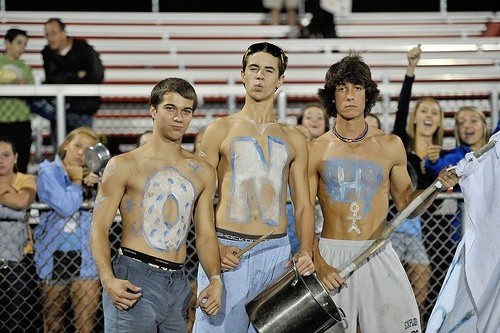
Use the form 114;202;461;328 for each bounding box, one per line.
209;274;223;286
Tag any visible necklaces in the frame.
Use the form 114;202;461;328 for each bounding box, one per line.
332;120;369;143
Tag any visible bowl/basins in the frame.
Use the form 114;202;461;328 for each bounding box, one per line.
84;143;111;173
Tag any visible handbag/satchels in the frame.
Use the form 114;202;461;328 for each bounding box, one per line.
52;250;81;280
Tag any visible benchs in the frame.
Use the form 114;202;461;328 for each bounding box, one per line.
0;7;500;150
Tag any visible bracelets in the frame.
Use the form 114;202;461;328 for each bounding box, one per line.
72;178;82;181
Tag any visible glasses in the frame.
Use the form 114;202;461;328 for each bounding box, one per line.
243;43;284;72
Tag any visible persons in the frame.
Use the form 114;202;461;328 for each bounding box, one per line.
40;18;106;150
422;106;500;251
386;130;431;333
89;77;223;333
263;0;304;25
33;126;101;333
391;42;456;333
192;42;315;333
0;29;36;174
0;138;45;333
308;49;462;333
296;104;331;243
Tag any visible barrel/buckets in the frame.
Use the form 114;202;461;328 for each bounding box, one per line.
246;256;348;333
